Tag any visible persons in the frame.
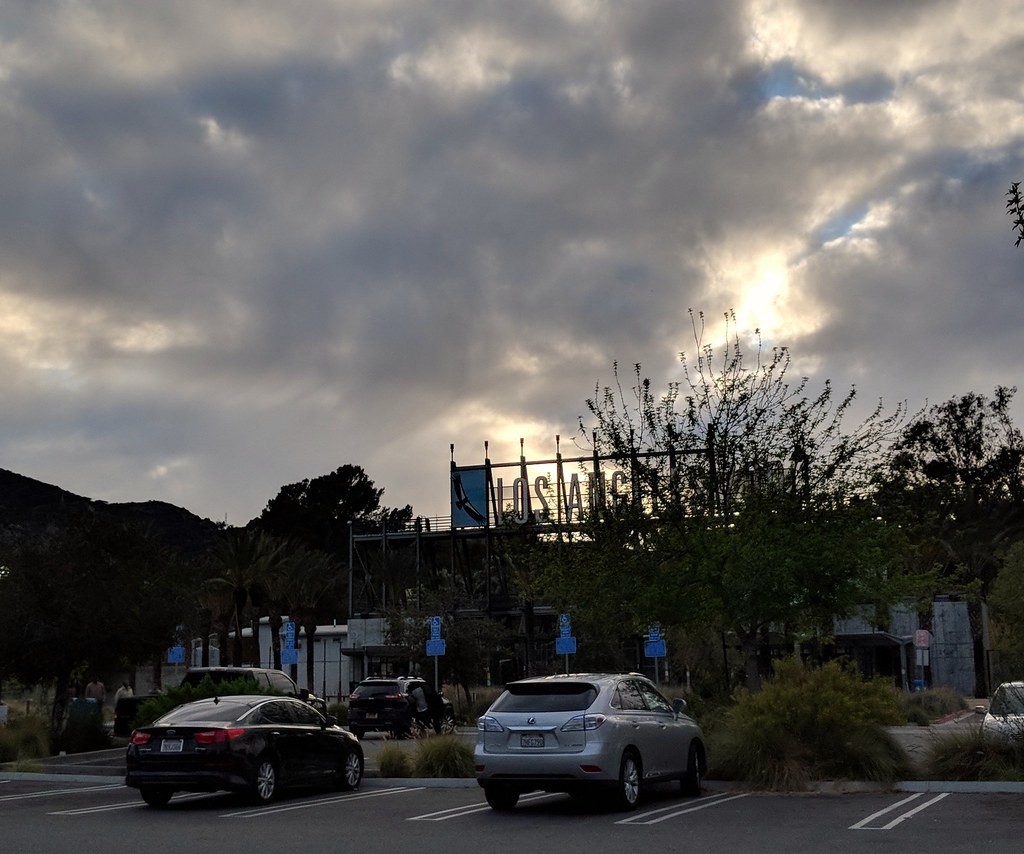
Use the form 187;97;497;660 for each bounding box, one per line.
85;674;107;710
112;680;134;714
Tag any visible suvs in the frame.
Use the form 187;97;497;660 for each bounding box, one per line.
473;670;708;813
347;676;456;739
978;680;1024;744
177;665;330;723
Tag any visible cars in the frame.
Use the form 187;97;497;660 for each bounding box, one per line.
123;693;364;808
